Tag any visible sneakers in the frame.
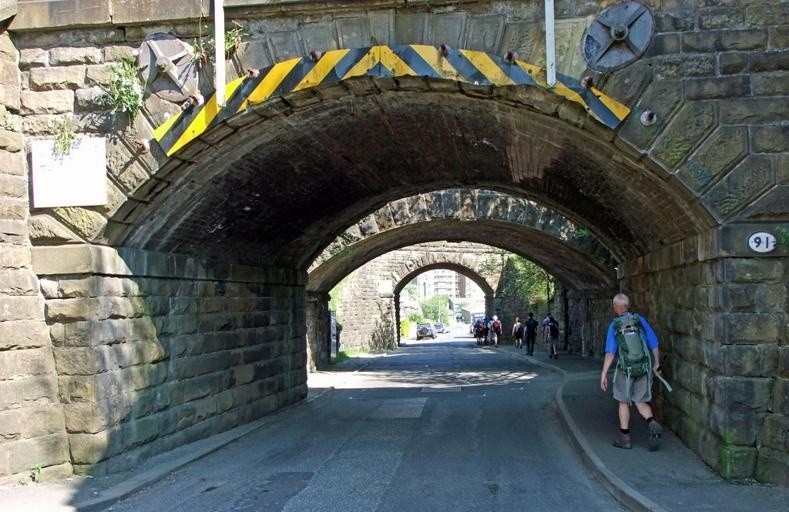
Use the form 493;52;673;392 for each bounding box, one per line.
646;418;663;452
610;429;633;449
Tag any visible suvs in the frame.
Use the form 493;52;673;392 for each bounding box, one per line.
416;324;437;339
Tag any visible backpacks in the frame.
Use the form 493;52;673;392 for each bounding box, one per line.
515;323;524;335
612;313;650;378
492;319;500;330
548;323;560;339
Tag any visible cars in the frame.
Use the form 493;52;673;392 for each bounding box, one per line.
435;323;448;333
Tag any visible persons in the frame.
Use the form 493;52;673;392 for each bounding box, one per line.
472;311;560;359
600;292;663;452
